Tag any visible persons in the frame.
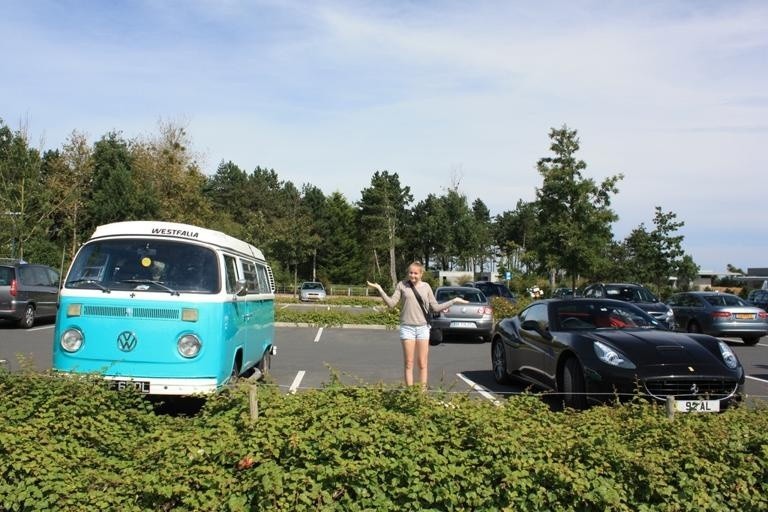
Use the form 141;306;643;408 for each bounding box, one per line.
366;260;471;390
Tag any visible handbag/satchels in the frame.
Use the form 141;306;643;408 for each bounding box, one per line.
430;328;442;345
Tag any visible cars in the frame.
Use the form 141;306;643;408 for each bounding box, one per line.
490;298;746;413
298;282;327;302
553;287;577;297
427;287;494;346
746;289;767;313
582;282;674;331
665;291;768;345
464;279;516;305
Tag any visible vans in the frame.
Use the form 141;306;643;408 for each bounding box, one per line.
0;262;63;329
51;221;277;397
